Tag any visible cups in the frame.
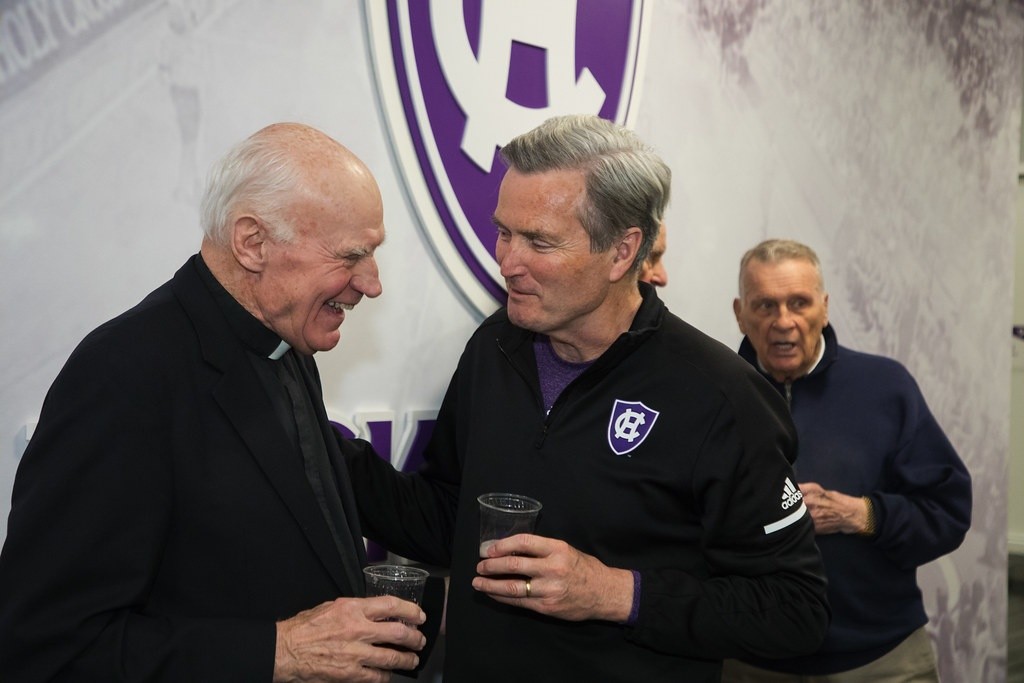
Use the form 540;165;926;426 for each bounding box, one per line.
478;491;542;580
363;566;429;653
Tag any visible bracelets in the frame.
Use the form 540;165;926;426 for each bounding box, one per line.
860;496;874;534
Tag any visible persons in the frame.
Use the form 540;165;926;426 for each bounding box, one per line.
0;123;428;683
330;115;830;683
735;241;973;683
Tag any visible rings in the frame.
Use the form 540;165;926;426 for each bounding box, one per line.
526;580;531;598
